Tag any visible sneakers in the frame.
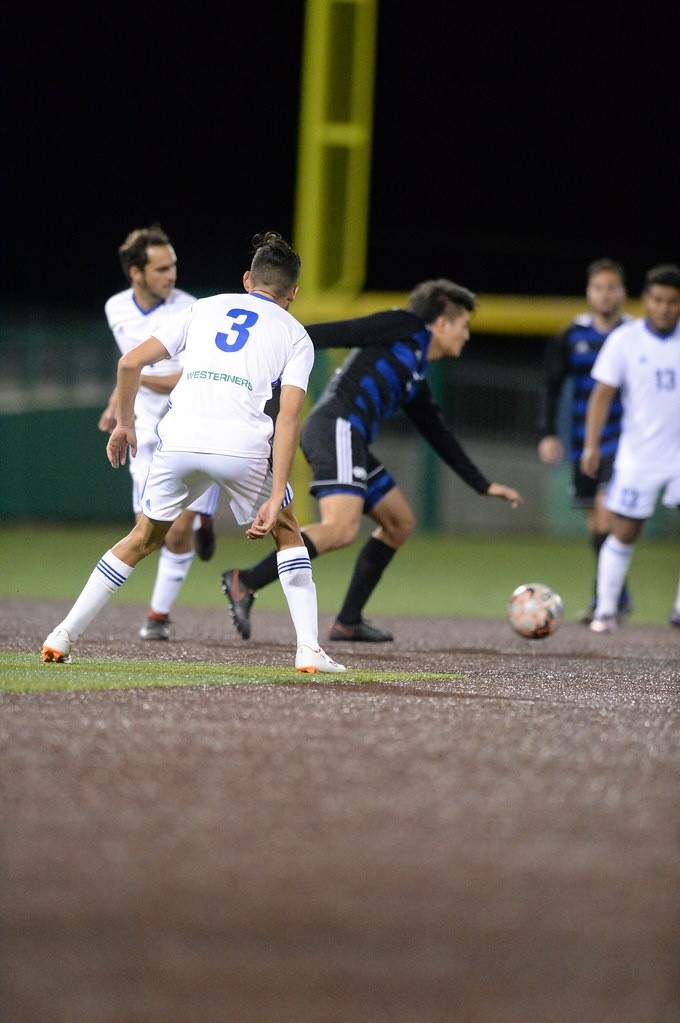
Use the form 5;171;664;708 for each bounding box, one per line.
330;616;393;642
194;515;214;562
40;627;74;662
295;643;347;675
140;616;170;641
222;568;255;640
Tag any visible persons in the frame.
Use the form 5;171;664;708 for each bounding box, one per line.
40;229;348;673
539;260;680;634
222;281;522;641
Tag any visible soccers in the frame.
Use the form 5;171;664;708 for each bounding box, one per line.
508;582;564;641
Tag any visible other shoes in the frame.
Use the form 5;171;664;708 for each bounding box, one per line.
589;612;617;633
580;593;629;627
